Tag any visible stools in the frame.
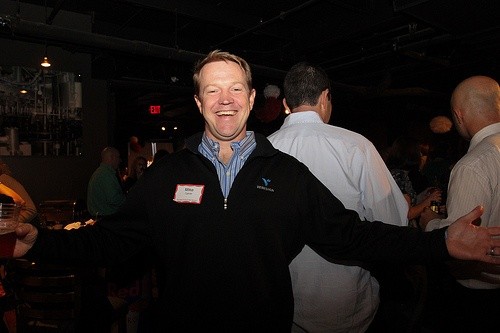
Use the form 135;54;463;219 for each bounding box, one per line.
39;191;81;226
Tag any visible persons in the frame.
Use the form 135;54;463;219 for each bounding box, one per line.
267;62;409;333
87;148;170;221
377;132;454;232
0;50;500;333
418;75;500;333
0;155;42;313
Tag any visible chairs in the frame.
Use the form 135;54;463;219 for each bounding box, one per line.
6;255;130;333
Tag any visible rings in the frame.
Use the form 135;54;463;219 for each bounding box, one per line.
491;248;494;255
